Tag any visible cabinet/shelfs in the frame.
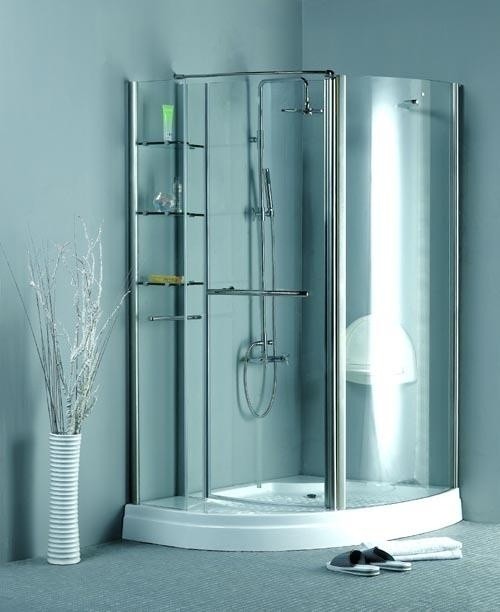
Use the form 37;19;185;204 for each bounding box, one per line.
137;141;206;287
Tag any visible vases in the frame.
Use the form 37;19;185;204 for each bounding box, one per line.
46;431;84;566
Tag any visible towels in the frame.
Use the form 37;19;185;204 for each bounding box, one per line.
376;535;464;561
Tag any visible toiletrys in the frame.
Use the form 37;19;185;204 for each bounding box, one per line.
161;103;175;141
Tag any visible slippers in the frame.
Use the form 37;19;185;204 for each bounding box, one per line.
326;549;381;578
359;547;412;572
